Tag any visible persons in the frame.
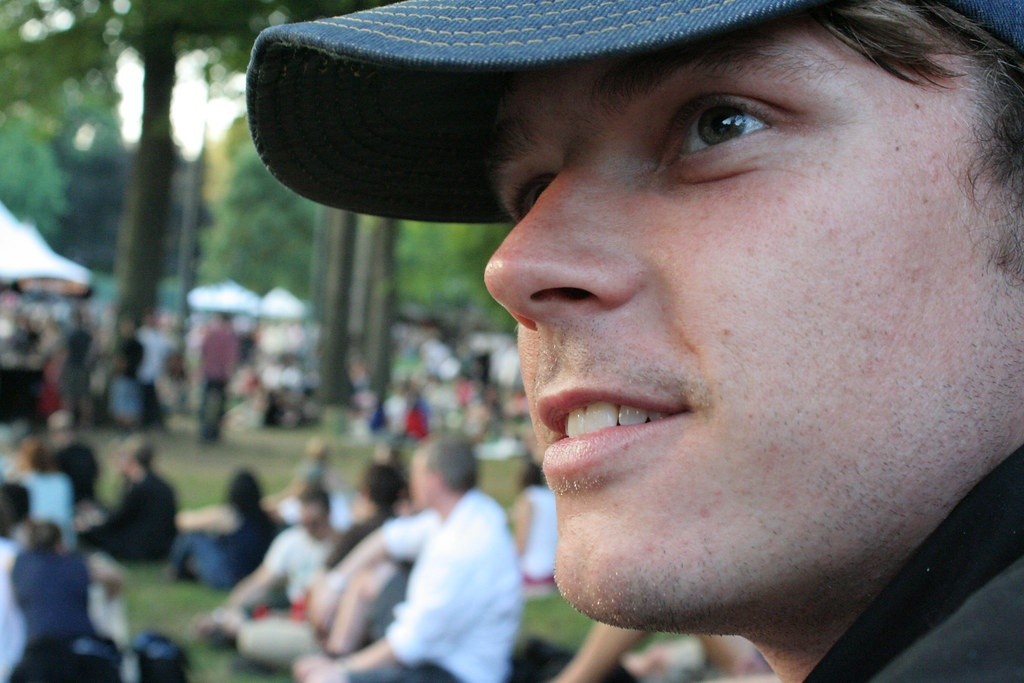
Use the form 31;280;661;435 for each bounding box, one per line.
245;0;1023;683
1;281;778;682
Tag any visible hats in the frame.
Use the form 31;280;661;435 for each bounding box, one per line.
246;0;1024;226
